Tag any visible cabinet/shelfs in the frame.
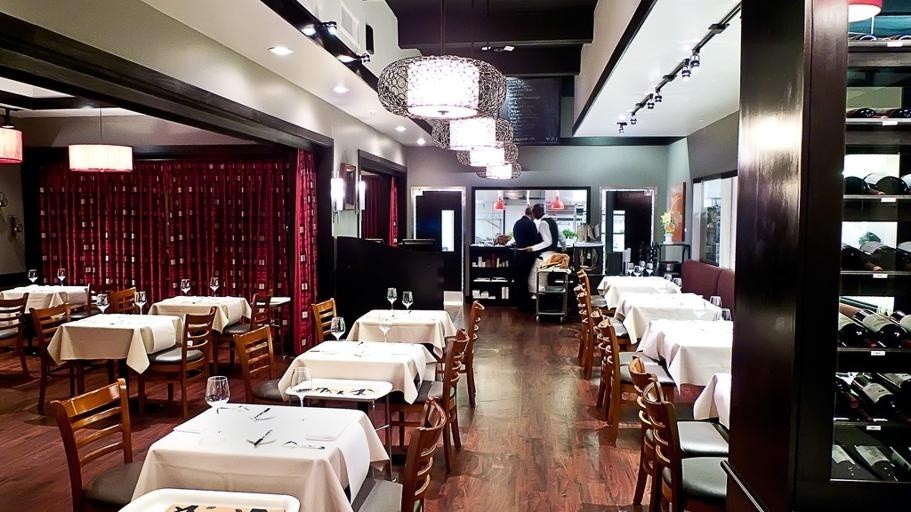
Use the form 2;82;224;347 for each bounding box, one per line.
470;243;605;307
650;241;691;279
791;0;911;512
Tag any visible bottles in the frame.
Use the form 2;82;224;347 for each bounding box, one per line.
842;166;909;193
827;440;909;483
839;224;909;275
838;292;909;349
832;374;910;423
848;104;910;125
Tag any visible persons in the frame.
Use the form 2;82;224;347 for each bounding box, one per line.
514;206;544;312
513;202;568;311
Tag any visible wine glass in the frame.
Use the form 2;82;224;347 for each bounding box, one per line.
663;273;682;300
690;294;732;332
386;287;397;316
205;376;230;433
379;319;391;348
626;261;653;282
56;268;66;289
28;270;39;290
401;291;413;317
330;317;345;349
209;277;219;299
95;294;109;320
134;292;147;319
181;279;190;300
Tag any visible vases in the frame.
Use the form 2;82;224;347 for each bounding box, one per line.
663;233;672;242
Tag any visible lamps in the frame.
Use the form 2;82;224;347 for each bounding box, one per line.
314;19;337;36
356;180;365;215
0;105;23;163
68;107;132;174
616;0;742;136
357;52;370;64
493;189;507;210
332;178;344;221
551;190;565;210
376;0;521;179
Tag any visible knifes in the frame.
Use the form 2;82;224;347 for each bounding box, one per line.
254;430;272;446
253;408;269;418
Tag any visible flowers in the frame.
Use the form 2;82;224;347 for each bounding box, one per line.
660;209;675;234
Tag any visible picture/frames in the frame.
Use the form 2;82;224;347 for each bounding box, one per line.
340;163;356;210
